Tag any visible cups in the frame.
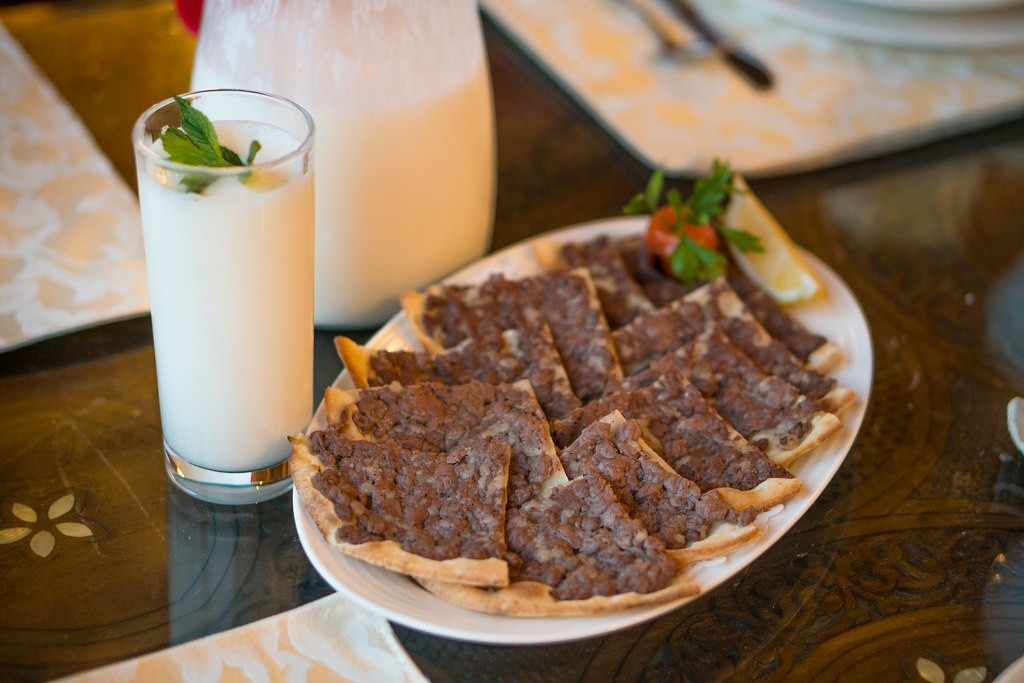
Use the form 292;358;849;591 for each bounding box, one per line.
169;492;295;649
133;88;315;509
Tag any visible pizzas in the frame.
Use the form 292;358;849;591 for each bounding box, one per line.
287;227;857;620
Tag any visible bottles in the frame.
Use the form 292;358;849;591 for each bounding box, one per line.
189;1;499;327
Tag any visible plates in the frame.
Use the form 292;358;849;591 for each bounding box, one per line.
292;214;875;645
476;0;1023;178
47;594;428;683
0;28;151;354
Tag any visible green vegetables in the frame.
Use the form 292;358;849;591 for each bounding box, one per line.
621;158;763;291
157;94;262;197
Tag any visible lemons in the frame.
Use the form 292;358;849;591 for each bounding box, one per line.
722;172;823;304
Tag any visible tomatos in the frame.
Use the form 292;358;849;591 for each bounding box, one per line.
649;207;720;268
